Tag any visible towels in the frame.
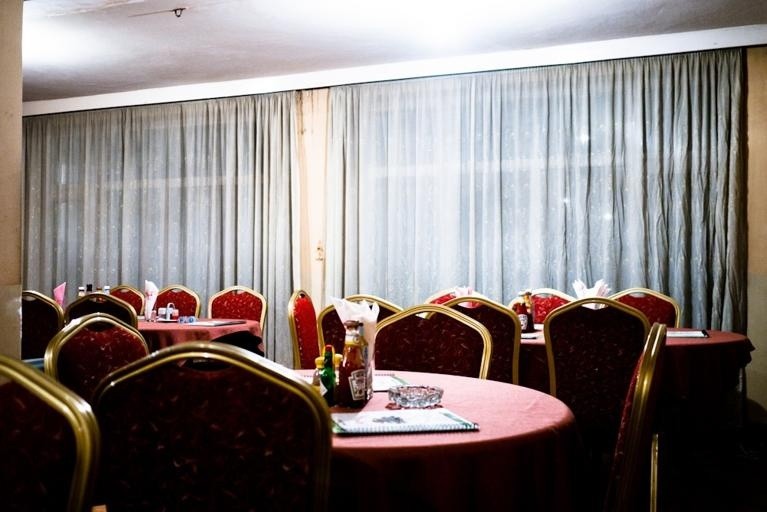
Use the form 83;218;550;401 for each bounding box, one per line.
573;279;610;309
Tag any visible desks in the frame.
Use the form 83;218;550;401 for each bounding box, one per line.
520;322;756;424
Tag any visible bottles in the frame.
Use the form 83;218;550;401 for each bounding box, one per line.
319;346;336;407
356;322;373;402
313;357;324;398
336;320;367;408
514;291;535;333
157;307;179;321
76;284;111;303
331;355;343;386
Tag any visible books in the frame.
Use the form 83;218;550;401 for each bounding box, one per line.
300;372;411;395
330;409;480;436
665;329;709;338
182;319;244;327
521;333;545;340
